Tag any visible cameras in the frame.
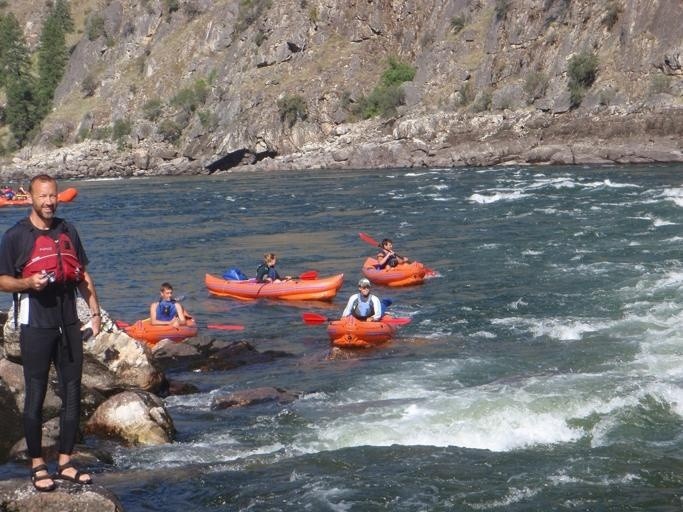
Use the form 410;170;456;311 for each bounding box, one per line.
40;269;56;283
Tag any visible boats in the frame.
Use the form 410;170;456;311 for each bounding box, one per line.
123;317;198;346
0;187;78;207
205;256;425;349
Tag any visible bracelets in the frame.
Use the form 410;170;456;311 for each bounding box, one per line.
93;313;100;317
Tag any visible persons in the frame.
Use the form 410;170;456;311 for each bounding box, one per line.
150;283;186;325
376;238;408;269
256;253;293;283
1;175;100;490
342;278;382;322
0;184;28;199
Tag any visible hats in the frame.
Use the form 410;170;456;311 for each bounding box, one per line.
358;278;371;288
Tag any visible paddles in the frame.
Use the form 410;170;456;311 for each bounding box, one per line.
357;230;437;276
114;320;245;331
234;271;317;284
302;312;411;327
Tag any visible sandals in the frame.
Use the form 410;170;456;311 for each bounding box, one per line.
31;463;56;492
53;461;93;485
